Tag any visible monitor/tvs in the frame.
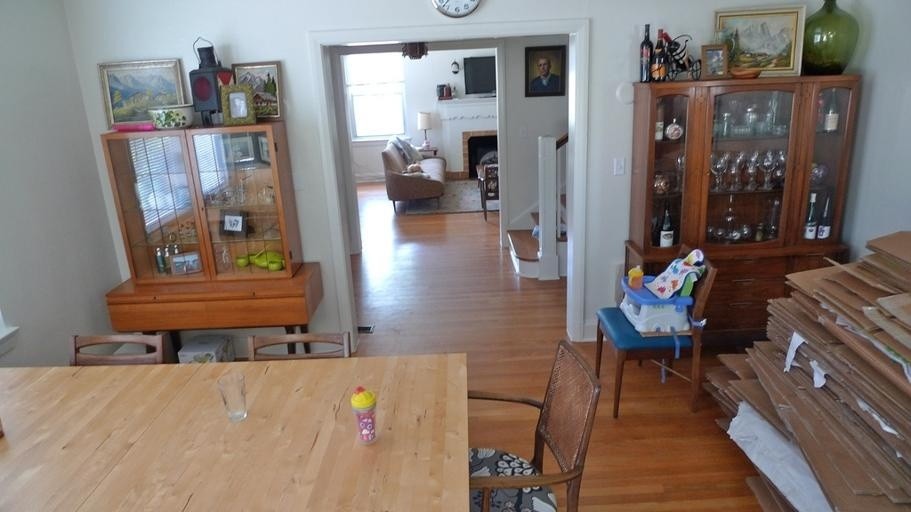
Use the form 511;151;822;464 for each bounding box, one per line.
463;57;496;97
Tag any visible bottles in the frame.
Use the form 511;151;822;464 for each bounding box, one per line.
154;245;180;273
803;189;831;239
656;98;665;140
726;207;734;232
816;88;841;133
351;384;377;444
657;198;674;247
438;85;451;97
712;106;786;138
641;23;693;83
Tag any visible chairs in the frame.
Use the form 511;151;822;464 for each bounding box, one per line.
247;331;350;361
480;164;500;221
68;330;171;366
467;338;602;512
595;244;718;418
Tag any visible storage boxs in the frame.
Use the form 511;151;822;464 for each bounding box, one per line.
177;334;236;363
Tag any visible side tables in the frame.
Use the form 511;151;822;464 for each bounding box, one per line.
414;147;438;156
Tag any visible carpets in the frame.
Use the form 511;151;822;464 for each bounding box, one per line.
406;180;500;214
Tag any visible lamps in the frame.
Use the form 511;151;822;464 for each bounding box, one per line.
417;112;433;148
452;61;459;74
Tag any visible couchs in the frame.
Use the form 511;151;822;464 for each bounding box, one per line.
381;137;447;212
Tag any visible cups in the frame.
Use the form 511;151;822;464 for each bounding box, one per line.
217;374;248;422
628;264;644;288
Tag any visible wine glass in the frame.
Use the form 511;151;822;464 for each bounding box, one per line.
711;150;788;193
674;152;685;190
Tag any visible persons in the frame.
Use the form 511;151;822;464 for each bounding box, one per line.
530;57;561;93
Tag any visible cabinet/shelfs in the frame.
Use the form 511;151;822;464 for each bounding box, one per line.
622;74;865;361
99;120;324;354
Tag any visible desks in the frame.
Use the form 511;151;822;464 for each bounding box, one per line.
0;353;471;512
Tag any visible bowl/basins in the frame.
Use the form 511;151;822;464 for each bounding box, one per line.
146;104;194;130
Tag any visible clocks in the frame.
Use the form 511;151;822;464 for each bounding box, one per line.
432;0;481;18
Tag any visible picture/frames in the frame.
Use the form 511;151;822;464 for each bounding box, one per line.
232;62;283;122
98;58;185;130
214;132;260;168
219;209;249;238
220;84;257;126
700;45;728;80
525;45;566;97
255;132;274;165
715;5;806;78
